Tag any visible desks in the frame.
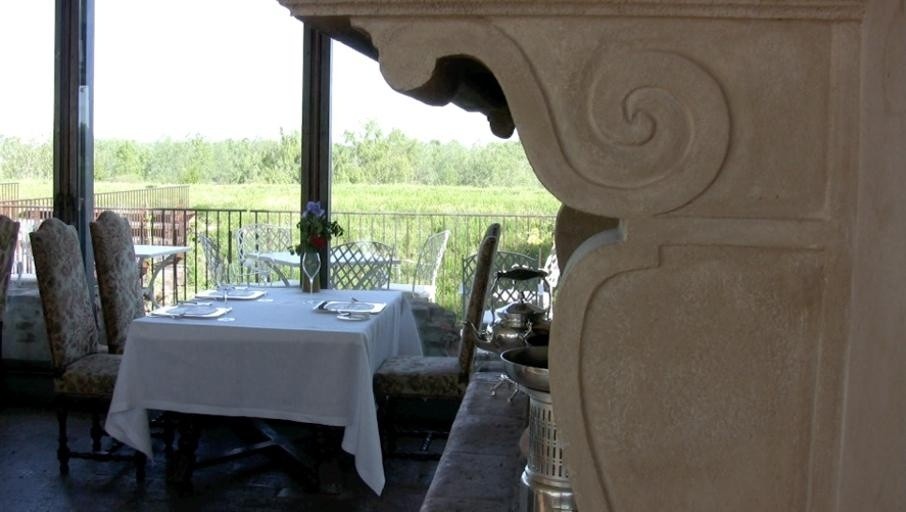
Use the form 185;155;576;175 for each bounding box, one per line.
250;251;402;288
134;245;192;308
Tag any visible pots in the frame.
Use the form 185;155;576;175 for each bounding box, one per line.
500;348;552;390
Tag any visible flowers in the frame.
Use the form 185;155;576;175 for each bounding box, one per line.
286;201;344;256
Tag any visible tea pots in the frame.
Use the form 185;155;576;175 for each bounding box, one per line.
461;269;551;353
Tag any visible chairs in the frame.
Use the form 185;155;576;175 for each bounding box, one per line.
236;224;300;286
462;245;542;325
90;211;147;353
29;219;173;490
373;222;500;477
0;216;20;334
330;241;394;290
199;234;274;286
382;230;450;303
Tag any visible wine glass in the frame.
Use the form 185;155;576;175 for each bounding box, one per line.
215;263;235;304
